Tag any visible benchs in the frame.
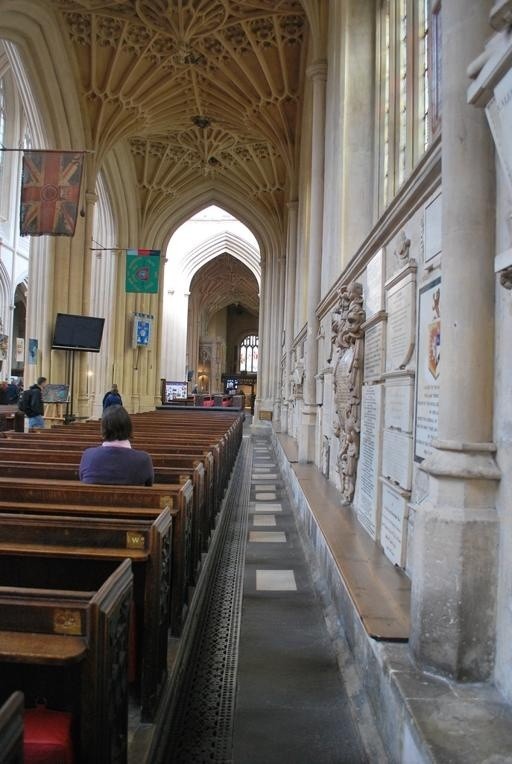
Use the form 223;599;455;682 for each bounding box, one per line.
0;410;245;764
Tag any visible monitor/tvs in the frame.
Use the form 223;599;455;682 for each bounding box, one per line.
52;312;105;354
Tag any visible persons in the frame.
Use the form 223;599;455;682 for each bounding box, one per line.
6;380;19;403
102;382;121;408
0;379;11;407
26;376;47;432
102;387;122;413
78;402;156;486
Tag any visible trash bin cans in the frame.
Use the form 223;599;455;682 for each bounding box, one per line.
203;397;215;407
221;396;233;407
14;412;24;432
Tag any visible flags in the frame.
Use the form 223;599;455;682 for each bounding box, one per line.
125;247;161;296
17;148;88;236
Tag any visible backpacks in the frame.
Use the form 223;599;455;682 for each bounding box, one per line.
17;390;33;411
